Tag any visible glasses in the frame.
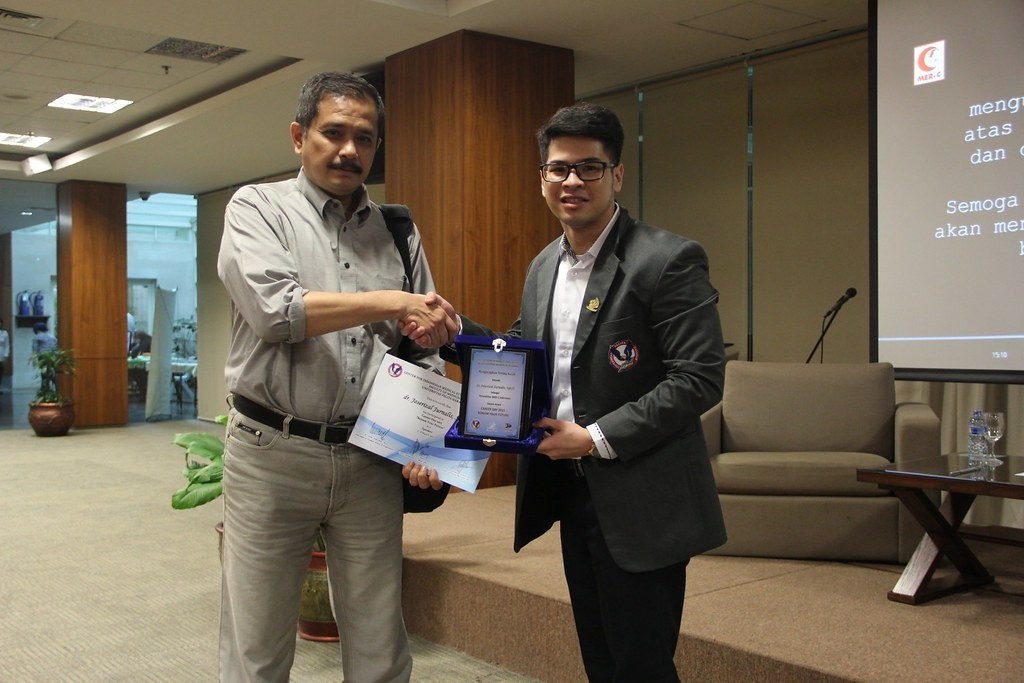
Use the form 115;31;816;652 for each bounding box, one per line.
539;160;614;183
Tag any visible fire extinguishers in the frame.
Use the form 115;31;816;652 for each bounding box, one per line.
16;290;31;316
29;290;46;316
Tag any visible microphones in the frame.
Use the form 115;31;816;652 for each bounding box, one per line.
824;287;857;318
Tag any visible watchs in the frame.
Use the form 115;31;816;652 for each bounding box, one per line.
589;439;600;456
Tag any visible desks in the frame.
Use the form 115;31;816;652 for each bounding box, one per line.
145;361;198;410
856;449;1024;604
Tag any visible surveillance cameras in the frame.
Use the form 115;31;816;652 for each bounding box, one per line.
139;191;151;201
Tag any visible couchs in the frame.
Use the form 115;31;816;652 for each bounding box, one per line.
698;361;941;563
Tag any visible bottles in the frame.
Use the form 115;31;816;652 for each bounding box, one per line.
968;409;987;466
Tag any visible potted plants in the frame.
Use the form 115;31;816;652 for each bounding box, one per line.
167;414;339;642
28;349;81;437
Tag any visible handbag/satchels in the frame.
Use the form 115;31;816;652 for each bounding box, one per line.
402;476;451;515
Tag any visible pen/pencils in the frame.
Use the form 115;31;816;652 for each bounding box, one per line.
400;275;408;291
536;427;552;438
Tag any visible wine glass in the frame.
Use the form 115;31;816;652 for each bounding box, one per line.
982;411;1005;467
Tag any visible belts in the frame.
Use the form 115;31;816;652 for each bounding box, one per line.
547;460;586;479
233;392;351;443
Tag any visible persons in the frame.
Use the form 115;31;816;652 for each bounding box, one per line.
129;331;152;389
31;323;57;392
398;104;727;682
216;73;443;683
